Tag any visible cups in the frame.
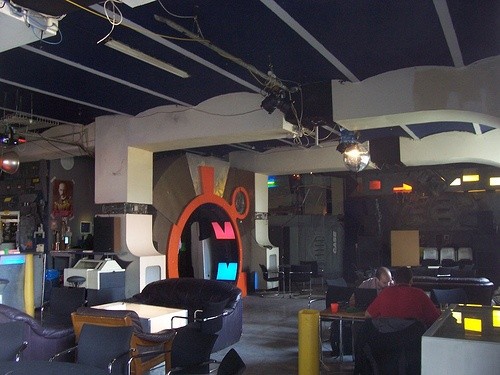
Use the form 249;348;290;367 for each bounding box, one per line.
330;304;338;313
5;249;8;255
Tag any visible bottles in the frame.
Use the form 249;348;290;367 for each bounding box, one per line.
338;302;351;314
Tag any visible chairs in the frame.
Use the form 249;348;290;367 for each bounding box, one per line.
258;263;284;299
0;320;29;363
354;315;426;375
48;322;136;372
419;245;476;270
41;286;88;327
127;328;218;375
308;285;357;312
69;305;176;375
430;287;468;305
299;261;324;293
192;347;250;375
287;265;313;299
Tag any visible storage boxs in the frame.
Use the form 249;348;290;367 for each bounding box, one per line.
91;302;188;333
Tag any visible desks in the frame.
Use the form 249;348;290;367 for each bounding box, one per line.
278;264;294;295
319;306;368;371
0;253;34;318
50;249;93;268
420;304;500;375
0;360;110;375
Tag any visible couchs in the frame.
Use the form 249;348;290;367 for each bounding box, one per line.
412;273;494;305
117;276;246;354
0;304;76;362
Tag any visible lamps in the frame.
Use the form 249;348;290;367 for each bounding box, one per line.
0;150;22;176
260;85;295;116
0;123;22;146
334;126;371;172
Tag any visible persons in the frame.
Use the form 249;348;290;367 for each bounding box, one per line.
349;266;394;307
53;183;73;218
365;266;442;329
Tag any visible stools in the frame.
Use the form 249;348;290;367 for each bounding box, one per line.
67;276;86;286
